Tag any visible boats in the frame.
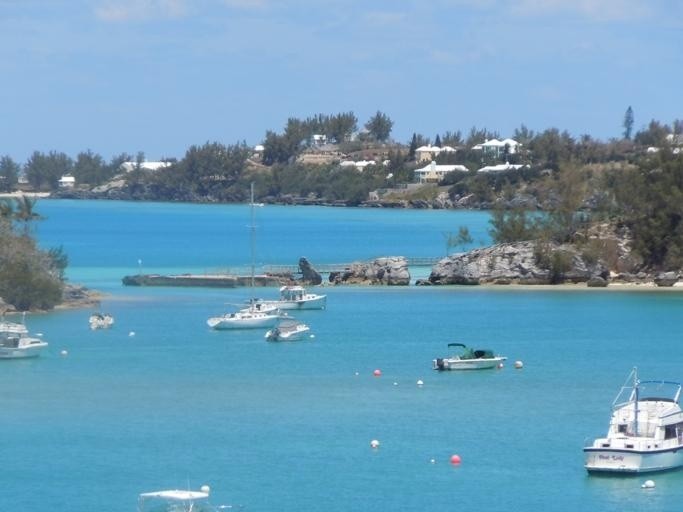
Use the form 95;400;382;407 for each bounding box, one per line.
205;280;328;342
432;354;508;371
581;364;683;475
0;320;48;360
